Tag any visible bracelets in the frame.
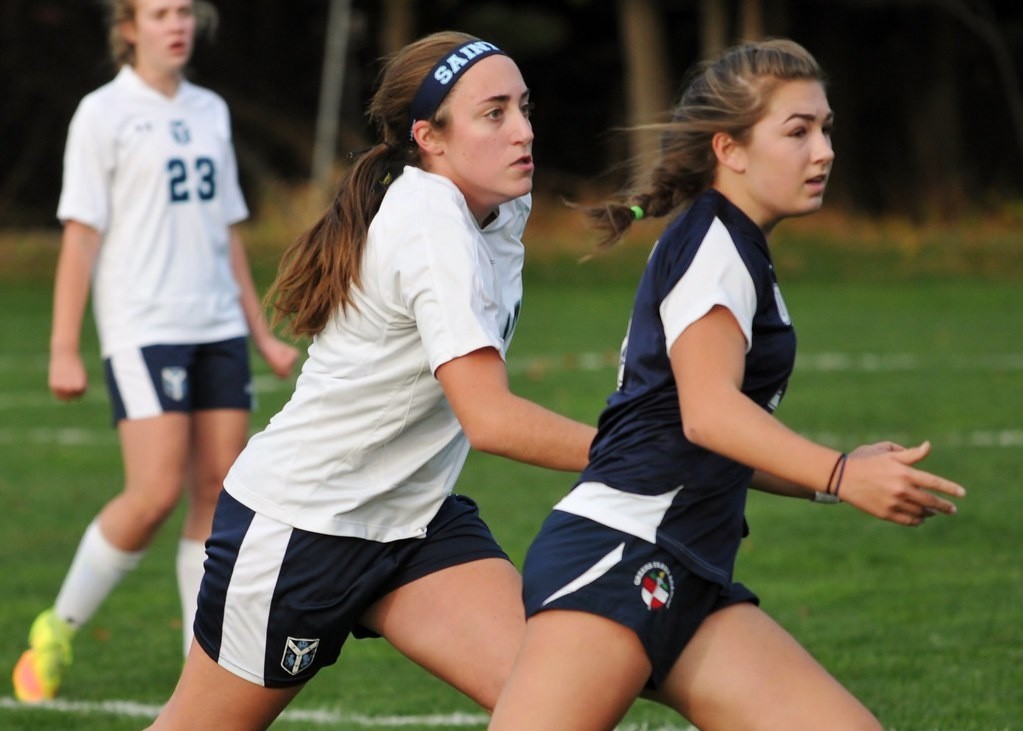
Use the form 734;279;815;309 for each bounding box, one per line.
811;490;840;504
826;453;846;497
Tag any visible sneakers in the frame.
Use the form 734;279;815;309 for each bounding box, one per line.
10;605;78;707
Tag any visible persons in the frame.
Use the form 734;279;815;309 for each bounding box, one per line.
14;0;300;705
148;32;598;731
490;42;966;731
